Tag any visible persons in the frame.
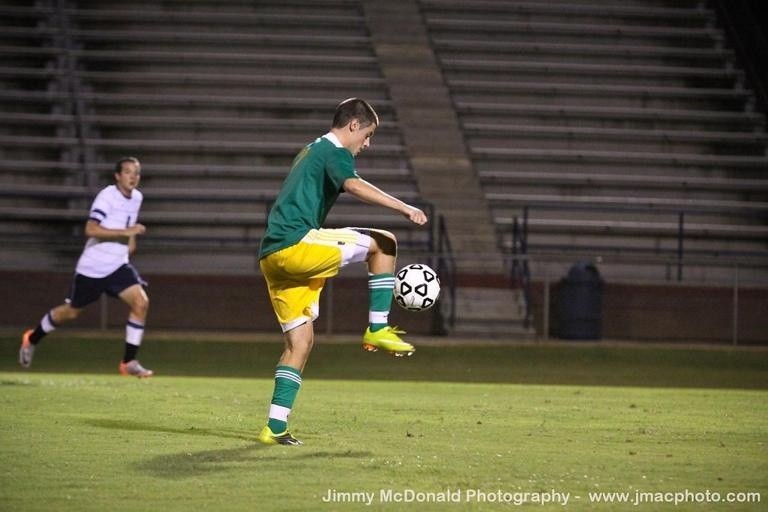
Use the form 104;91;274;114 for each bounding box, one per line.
16;155;153;376
254;98;428;447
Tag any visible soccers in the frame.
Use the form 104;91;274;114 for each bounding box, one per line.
393;264;440;312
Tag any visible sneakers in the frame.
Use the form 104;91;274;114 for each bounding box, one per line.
258;424;303;446
18;328;37;368
118;359;153;378
363;325;416;356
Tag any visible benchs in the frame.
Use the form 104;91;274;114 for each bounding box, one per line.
0;2;768;294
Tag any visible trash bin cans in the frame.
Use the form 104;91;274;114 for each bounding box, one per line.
558;262;606;340
